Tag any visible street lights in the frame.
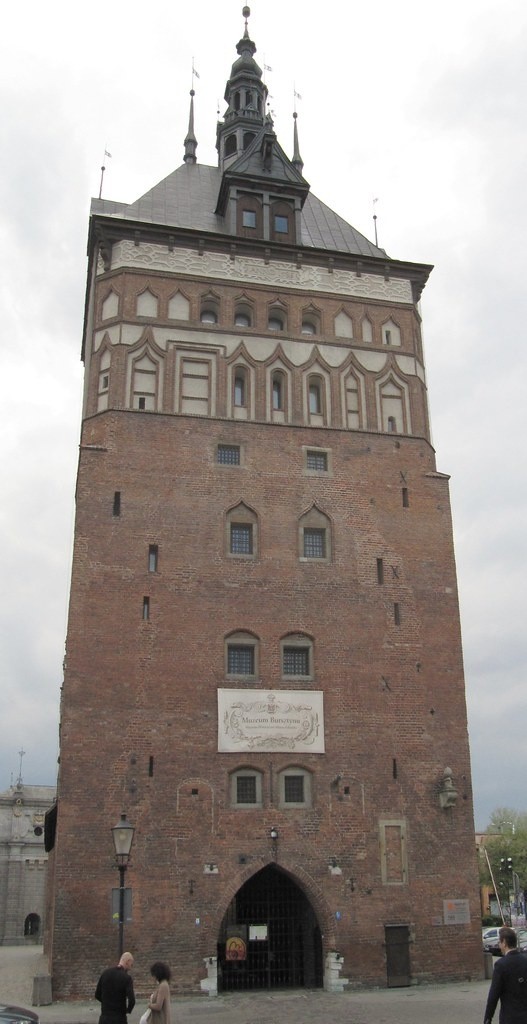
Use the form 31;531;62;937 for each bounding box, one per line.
500;858;513;926
110;812;135;960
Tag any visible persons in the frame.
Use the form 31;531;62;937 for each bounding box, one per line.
483;926;527;1024
140;961;172;1024
94;951;136;1024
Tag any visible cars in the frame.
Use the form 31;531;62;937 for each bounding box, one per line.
0;1003;39;1024
482;925;527;956
